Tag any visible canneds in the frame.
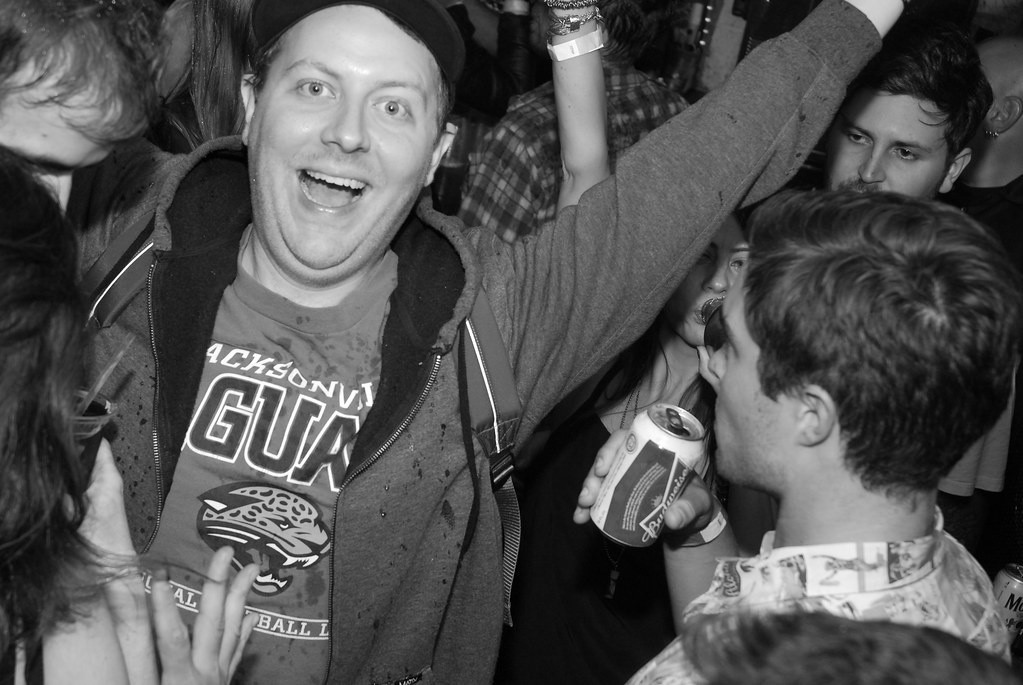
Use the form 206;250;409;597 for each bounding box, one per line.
994;563;1023;648
589;403;706;547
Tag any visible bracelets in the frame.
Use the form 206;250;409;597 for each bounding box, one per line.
547;8;603;36
680;494;727;547
547;24;604;61
543;0;599;10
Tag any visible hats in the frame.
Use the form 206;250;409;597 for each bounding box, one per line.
253;1;466;84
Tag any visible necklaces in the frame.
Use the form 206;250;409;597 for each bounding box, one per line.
619;378;643;430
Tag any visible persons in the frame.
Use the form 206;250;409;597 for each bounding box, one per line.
0;0;1023;685
